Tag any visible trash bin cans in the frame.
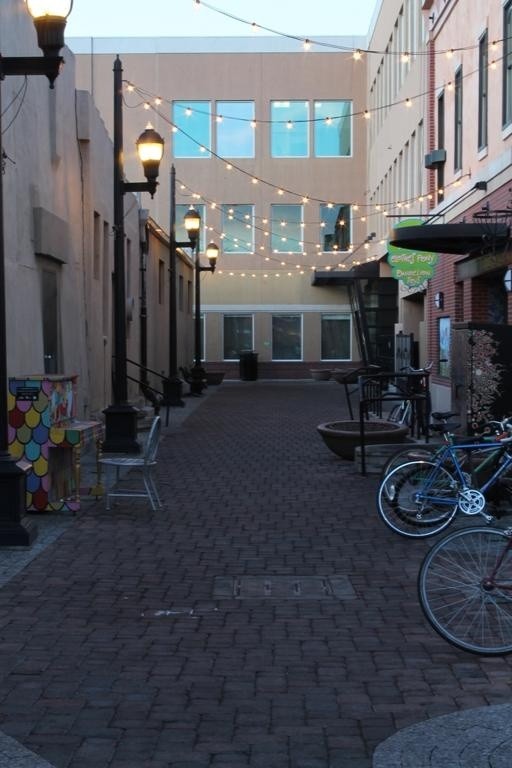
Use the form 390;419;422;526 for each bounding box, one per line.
240;350;259;381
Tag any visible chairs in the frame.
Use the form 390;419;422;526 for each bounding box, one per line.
97;414;163;510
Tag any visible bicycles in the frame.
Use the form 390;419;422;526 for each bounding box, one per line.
387;358;449;444
376;410;512;539
417;524;512;656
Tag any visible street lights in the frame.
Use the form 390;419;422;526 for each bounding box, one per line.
0;0;74;550
102;118;165;458
159;204;201;410
190;242;218;397
